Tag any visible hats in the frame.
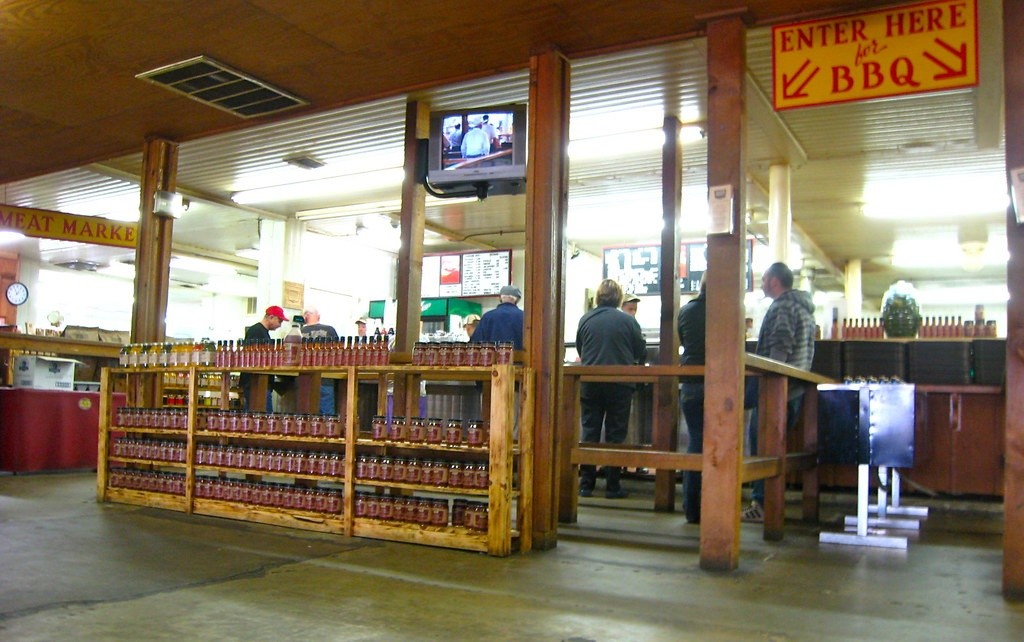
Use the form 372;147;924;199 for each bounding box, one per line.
463;314;481;328
266;306;289;321
355;317;372;324
622;293;640;304
499;285;523;299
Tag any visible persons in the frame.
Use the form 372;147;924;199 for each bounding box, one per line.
239;305;388;417
440;113;503;169
574;279;647;500
739;262;815;522
676;269;710;525
463;286;524;490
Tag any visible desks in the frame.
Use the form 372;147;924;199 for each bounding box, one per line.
0;387;126;475
562;352;834;544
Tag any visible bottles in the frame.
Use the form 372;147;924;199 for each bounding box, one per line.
950;316;956;337
860;318;865;339
343;336;359;366
234;339;286;367
372;336;389;365
871;318;877;338
163;372;240;408
284;316;304;366
865;318;871;338
924;317;930;337
943;317;950;337
848;319;854;338
358;336;374;366
119;341;195;368
931;317;936;337
215;340;234;367
300;337;329;366
877;318;883;338
918;317;923;337
831;319;838;340
192;341;215;366
841;319;847;338
937;317;943;337
328;336;345;366
956;316;963;337
854;319;860;338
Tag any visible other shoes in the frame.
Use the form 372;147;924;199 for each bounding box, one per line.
605;488;629;499
579;489;593;497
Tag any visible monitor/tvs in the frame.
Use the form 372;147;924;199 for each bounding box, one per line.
428;103;529;196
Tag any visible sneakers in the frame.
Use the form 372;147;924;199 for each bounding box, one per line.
741;499;765;522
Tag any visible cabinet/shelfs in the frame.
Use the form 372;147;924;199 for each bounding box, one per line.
94;366;532;556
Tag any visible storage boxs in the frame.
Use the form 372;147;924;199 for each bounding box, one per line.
13;353;85;391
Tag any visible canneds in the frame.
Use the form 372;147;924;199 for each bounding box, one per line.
369;415;484;449
412;341;514;366
108;405;362;515
355;455;489;489
354;490;488;532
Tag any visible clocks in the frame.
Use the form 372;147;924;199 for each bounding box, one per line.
6;281;28;305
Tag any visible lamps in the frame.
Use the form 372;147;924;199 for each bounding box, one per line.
295;194;479;223
232;166;405;205
